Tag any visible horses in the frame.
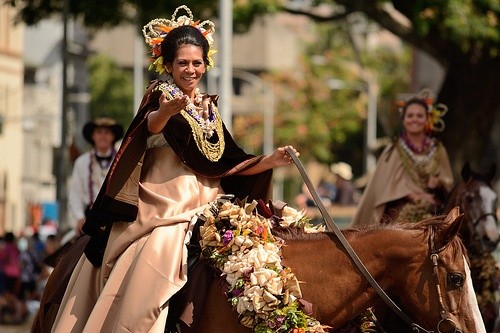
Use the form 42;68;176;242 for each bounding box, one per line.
30;206;477;333
380;163;500;333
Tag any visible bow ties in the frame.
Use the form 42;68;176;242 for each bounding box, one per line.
94;152;112;162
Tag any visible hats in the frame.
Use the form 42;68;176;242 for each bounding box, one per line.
82;115;124;147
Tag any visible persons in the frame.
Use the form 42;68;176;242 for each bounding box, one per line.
0;114;125;333
295;159;356;206
49;5;300;333
349;91;455;224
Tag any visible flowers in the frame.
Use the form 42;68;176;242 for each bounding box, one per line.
195;197;334;333
142;5;218;75
394;96;441;131
